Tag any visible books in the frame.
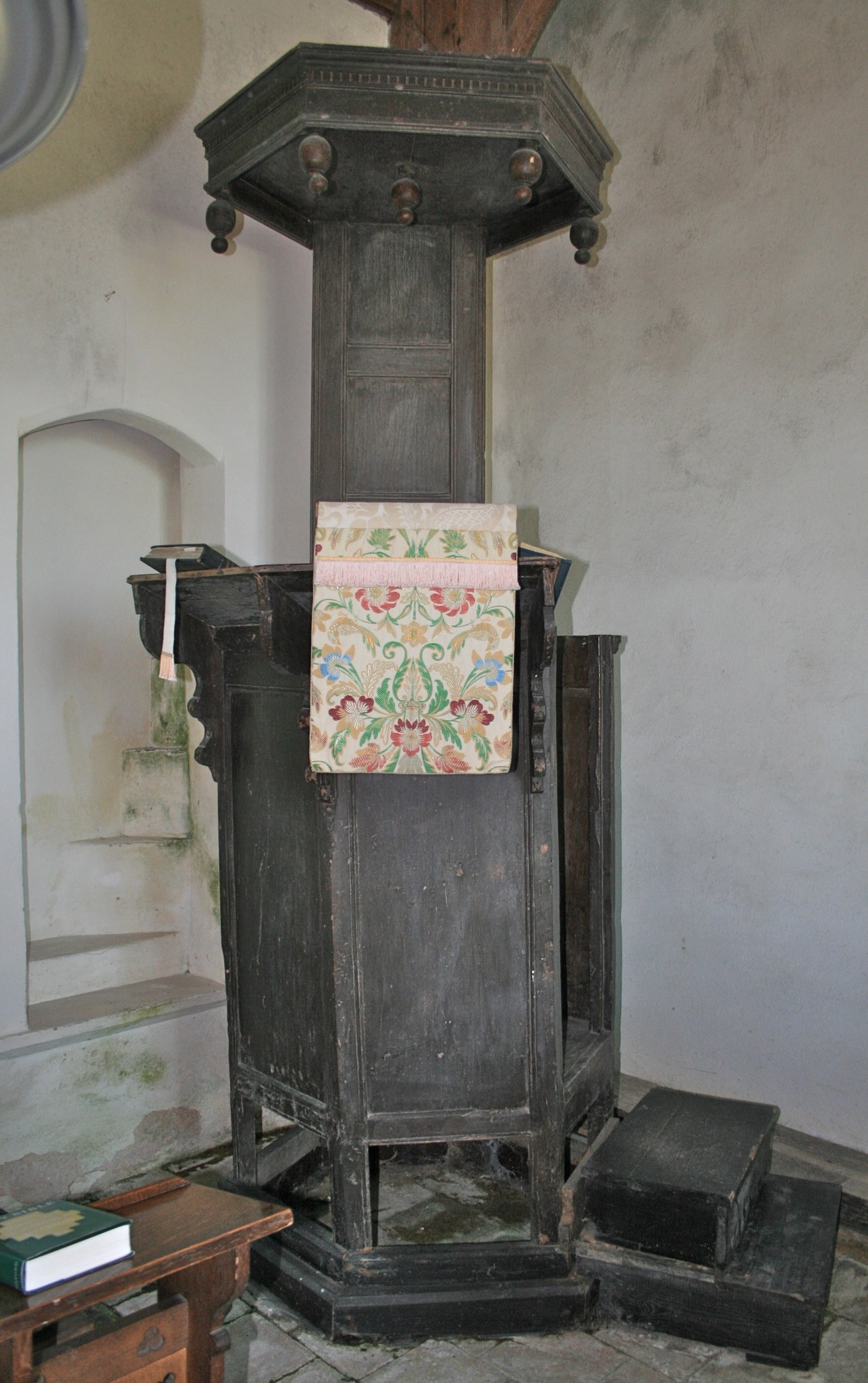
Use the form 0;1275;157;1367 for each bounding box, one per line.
0;1198;136;1295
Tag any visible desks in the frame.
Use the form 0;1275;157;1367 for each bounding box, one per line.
0;1176;297;1383
